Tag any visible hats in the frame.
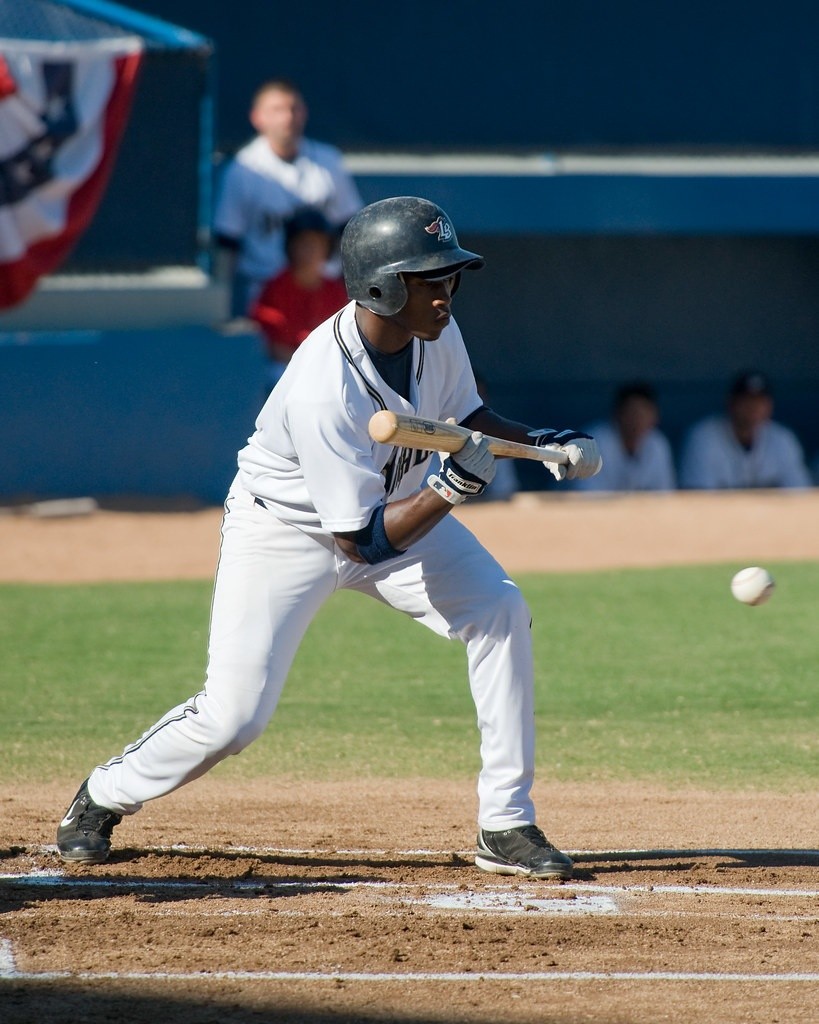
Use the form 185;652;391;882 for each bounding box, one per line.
730;369;774;397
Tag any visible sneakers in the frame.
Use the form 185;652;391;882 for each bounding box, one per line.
55;776;125;864
474;824;575;879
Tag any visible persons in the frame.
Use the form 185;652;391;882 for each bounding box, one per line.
58;193;580;883
466;377;816;503
208;83;370;366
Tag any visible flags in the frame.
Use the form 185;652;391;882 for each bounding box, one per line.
1;32;140;316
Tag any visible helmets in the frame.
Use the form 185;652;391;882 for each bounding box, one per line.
340;197;486;318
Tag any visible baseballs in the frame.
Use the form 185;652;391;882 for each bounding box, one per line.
729;566;775;605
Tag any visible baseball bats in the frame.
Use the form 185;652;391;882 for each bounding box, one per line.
368;410;568;465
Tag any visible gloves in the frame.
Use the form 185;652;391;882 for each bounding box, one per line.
539;428;603;482
425;417;501;506
